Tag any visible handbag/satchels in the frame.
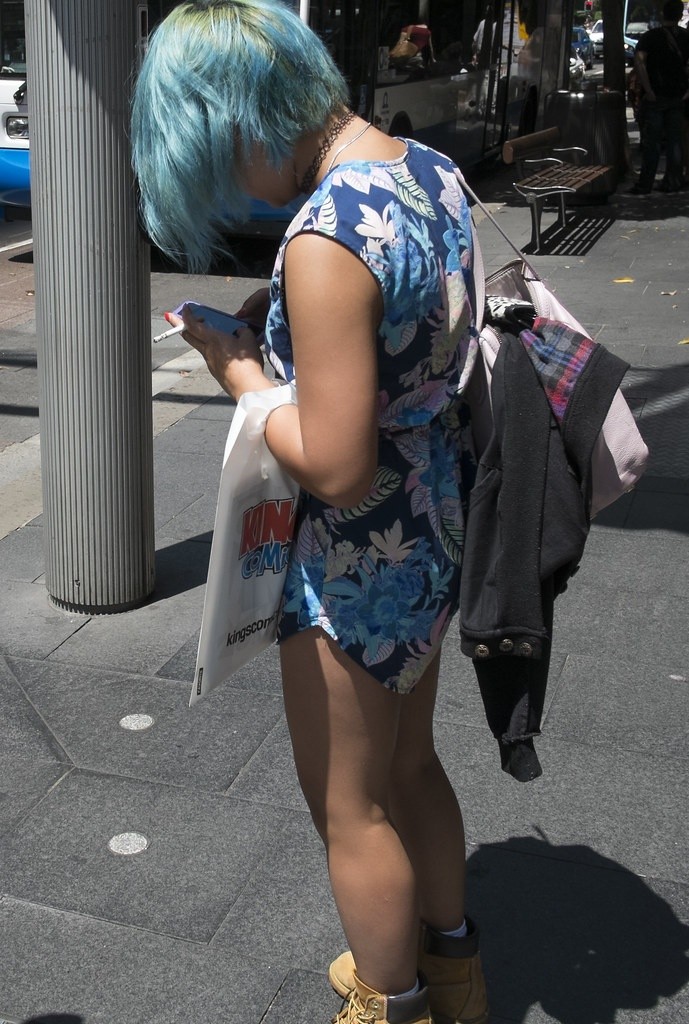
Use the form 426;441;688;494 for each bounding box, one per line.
187;380;299;705
452;168;649;521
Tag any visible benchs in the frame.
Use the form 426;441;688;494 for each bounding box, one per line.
502;126;614;253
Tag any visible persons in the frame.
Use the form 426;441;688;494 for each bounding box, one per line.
623;0;689;195
471;5;498;71
398;11;440;81
129;0;491;1024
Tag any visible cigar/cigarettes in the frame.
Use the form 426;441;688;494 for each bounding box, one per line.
153;316;205;343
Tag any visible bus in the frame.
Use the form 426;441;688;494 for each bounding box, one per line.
0;0;506;207
624;0;689;67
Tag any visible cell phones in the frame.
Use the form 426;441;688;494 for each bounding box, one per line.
173;301;266;347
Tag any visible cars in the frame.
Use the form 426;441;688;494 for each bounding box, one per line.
572;28;596;70
589;19;603;60
570;45;586;84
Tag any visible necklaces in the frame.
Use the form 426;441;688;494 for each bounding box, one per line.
327;122;372;173
298;109;357;195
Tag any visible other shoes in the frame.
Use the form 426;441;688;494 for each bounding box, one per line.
329;917;490;1024
624;186;651;194
329;968;434;1024
654;182;679;192
